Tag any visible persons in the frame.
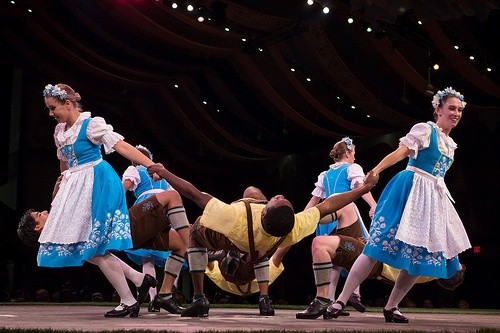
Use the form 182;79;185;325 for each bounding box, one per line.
303;138;378;312
37;83;162;318
18;178;191;315
323;87;472;323
147;162;377;317
120;145;188;314
295;194;466;321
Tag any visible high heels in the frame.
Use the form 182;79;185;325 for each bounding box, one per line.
136;273;159;306
104;302;140;318
181;297;210;317
148;300;160;312
338;312;350;316
296;298;328;319
346;292;366;313
326;300;345;318
383;306;409;323
259;297;275;316
152;294;186;314
